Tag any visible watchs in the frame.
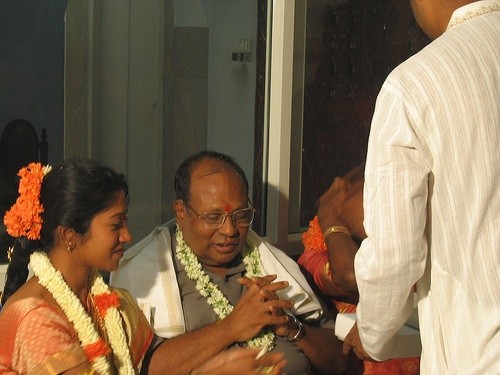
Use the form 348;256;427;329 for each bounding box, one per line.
278;315;304;343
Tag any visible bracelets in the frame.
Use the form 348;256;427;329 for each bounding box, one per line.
323;226;351;241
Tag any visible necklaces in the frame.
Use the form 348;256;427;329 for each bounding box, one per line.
30;251;135;375
176;225;275;359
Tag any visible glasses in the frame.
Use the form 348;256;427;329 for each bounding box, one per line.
181;197;255;230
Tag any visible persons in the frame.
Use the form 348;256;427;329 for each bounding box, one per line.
110;151;343;375
343;0;499;375
297;161;368;301
0;157;287;375
